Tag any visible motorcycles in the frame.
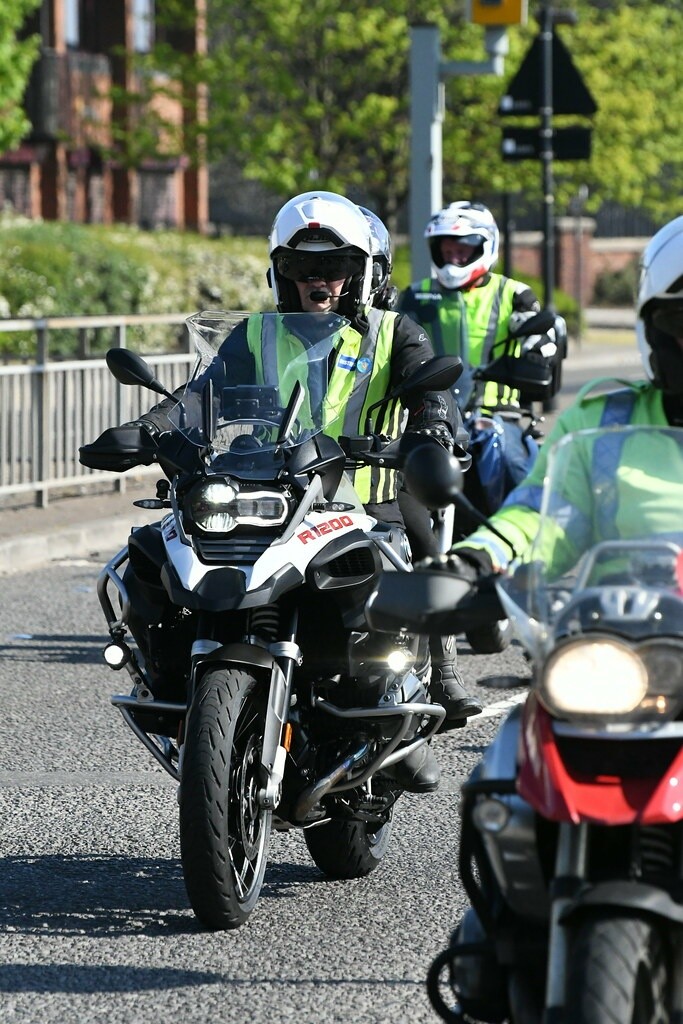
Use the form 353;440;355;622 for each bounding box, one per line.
382;310;557;660
368;418;683;1024
76;344;467;933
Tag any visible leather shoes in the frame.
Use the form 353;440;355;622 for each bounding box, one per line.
375;735;442;792
429;660;483;724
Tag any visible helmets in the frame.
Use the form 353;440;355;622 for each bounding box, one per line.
424;200;499;289
634;214;683;389
269;190;373;313
355;205;393;303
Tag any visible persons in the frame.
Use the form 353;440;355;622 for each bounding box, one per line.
358;205;483;721
114;190;461;794
416;214;682;598
396;198;560;516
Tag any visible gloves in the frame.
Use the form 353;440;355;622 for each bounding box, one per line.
120;418;160;439
405;420;455;447
414;553;479;582
522;351;550;368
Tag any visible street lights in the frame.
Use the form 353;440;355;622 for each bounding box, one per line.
535;0;578;316
